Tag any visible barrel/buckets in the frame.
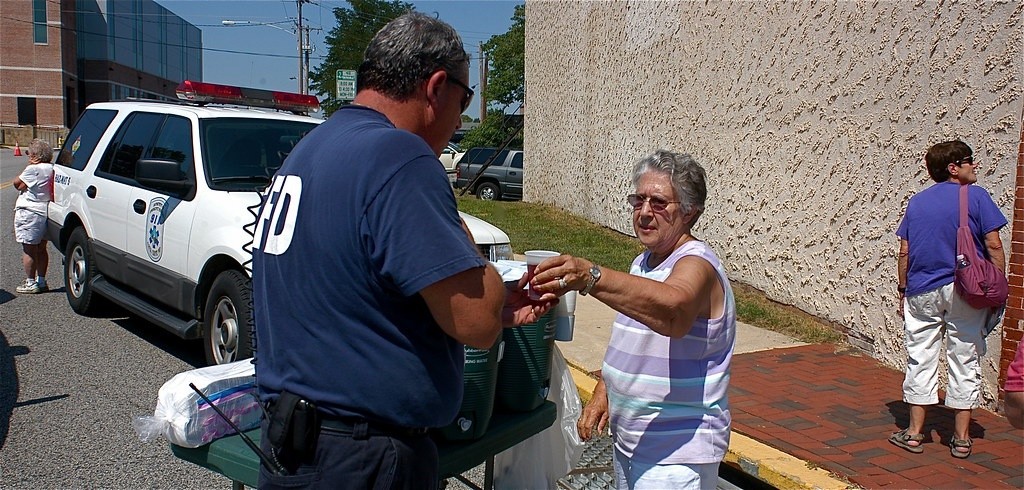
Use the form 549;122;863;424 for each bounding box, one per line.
500;302;557;412
434;328;504;441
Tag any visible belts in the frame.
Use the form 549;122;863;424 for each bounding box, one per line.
317;417;427;439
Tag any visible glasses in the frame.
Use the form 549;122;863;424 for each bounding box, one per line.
627;194;680;213
946;157;973;171
25;150;32;155
426;69;475;114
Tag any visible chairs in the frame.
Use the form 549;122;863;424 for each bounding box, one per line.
216;138;269;177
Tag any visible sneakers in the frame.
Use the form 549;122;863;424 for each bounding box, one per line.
16;280;40;293
35;280;49;291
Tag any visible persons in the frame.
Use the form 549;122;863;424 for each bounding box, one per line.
251;12;560;490
888;140;1008;458
13;139;55;293
530;149;736;490
1004;336;1024;432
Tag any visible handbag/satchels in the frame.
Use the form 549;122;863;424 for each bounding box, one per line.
954;184;1008;310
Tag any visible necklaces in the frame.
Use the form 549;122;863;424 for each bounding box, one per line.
350;102;377;111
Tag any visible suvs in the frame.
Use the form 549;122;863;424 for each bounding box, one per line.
46;99;518;369
452;147;524;202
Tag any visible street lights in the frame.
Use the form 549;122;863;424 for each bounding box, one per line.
221;20;304;94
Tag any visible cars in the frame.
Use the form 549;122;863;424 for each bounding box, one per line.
438;142;468;171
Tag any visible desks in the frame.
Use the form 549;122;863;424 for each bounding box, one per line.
170;400;558;490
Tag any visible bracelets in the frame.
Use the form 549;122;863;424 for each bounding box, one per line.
898;285;906;294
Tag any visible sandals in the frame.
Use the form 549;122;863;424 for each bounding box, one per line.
888;427;925;453
947;435;974;458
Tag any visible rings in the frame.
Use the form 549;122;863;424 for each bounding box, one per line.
532;308;535;313
558;278;567;289
578;418;582;421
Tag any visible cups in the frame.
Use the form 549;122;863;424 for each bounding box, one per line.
524;250;562;302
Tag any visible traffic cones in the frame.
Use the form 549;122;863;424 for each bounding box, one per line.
14;141;22;156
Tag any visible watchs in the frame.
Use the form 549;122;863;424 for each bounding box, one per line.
579;263;602;296
